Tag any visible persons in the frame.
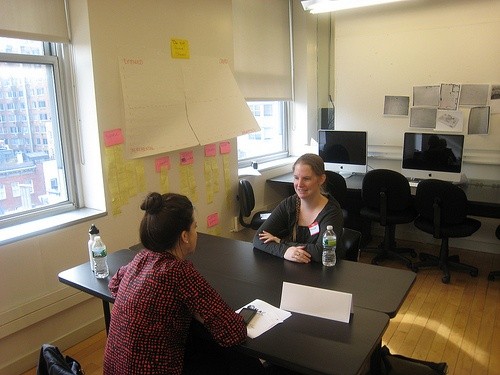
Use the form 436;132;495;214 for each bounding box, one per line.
102;191;248;375
252;153;345;264
422;135;457;169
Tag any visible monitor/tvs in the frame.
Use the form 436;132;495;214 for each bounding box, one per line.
400;131;465;183
318;130;368;179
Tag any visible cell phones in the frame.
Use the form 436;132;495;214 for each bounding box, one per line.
238;308;257;324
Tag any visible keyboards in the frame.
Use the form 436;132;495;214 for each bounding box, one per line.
409;181;418;188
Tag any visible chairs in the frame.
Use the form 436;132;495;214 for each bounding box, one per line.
37;344;84;375
414;179;482;284
239;179;272;230
323;171;360;225
361;169;413;272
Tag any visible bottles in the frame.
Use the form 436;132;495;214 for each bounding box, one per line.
321;225;337;266
92;236;109;278
88;224;100;271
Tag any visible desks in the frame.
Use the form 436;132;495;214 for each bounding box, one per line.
58;232;416;375
265;171;500;218
